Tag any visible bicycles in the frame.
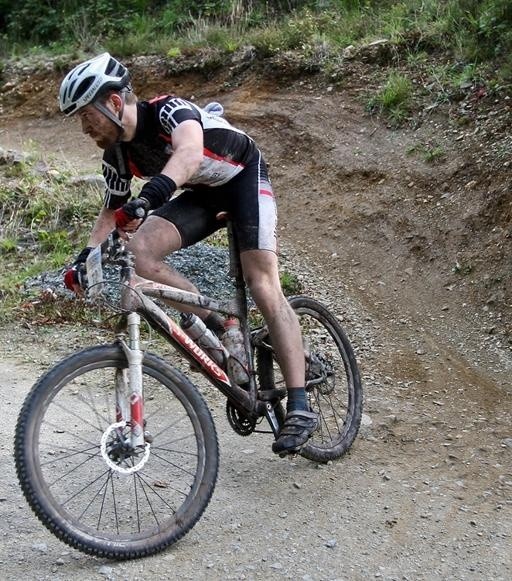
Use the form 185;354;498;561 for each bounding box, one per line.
14;208;366;560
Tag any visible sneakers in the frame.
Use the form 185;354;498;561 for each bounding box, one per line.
269;411;320;454
187;360;201;375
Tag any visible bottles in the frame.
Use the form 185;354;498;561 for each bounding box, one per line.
178;310;231;368
220;319;258;390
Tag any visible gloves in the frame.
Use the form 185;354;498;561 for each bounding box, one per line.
65;259;90;294
113;199;150;239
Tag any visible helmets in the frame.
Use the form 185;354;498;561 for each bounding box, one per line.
58;51;132;120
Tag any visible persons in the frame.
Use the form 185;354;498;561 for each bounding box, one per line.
58;52;321;455
